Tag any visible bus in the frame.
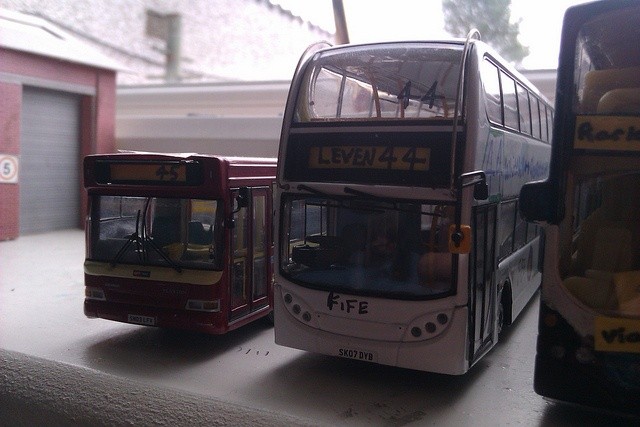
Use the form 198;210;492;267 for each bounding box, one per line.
82;150;275;339
519;0;640;420
275;27;555;377
